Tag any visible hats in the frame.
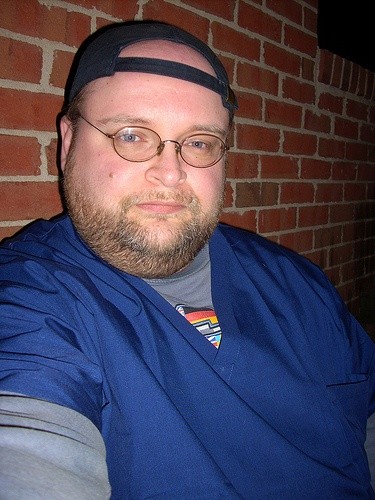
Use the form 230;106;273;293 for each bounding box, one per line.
67;19;240;111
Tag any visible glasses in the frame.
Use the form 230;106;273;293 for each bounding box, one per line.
77;109;230;169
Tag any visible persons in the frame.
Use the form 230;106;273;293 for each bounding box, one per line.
0;20;374;500
